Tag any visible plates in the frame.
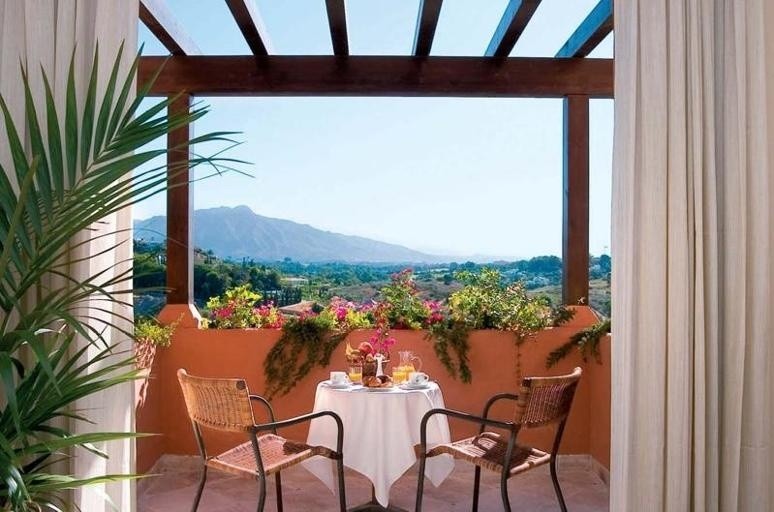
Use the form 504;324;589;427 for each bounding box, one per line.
403;383;429;390
324;381;353;388
365;385;393;391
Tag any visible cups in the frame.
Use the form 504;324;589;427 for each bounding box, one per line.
349;367;362;383
327;372;348;383
410;372;428;385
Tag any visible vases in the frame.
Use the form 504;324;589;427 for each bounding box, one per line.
361;356;391;378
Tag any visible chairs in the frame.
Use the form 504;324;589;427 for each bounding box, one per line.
174;366;348;512
410;366;584;512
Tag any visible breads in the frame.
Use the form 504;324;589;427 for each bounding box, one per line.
363;375;392;388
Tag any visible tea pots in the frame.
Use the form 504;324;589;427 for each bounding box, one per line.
397;350;421;380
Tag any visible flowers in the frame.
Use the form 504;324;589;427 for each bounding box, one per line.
358;327;397;362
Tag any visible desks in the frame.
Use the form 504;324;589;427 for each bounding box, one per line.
301;376;455;512
